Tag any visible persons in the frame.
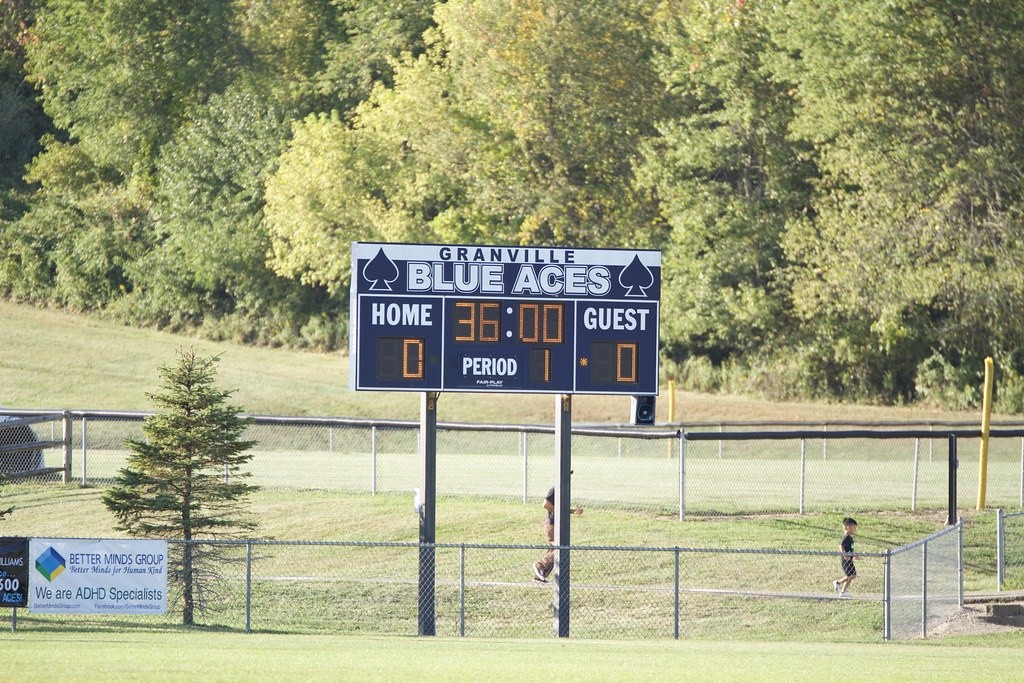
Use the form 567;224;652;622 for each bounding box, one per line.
832;518;858;597
533;470;583;583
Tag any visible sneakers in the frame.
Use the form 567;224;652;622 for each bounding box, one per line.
532;562;545;581
532;575;550;584
840;592;851;597
834;580;839;591
